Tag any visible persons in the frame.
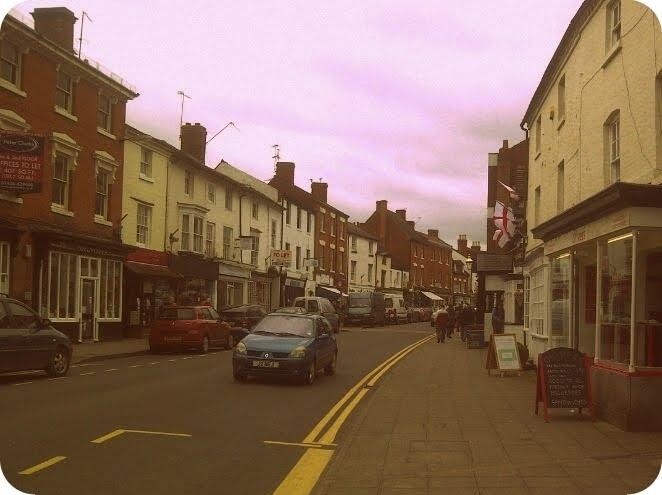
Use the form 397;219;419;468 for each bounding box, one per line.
145;296;216;324
492;303;503;333
430;301;482;344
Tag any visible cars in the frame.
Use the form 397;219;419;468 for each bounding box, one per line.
147;304;234;352
221;304;270;331
226;311;338;386
431;311;439;325
276;306;308;315
0;292;73;377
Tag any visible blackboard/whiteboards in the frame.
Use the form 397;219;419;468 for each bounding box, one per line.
538;347;593;408
492;333;523;372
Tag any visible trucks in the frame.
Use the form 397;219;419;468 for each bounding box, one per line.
344;291;385;327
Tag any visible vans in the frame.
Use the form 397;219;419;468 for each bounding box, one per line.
292;296;340;333
384;293;409;324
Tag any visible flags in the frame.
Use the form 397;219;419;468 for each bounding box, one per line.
492;181;519;248
